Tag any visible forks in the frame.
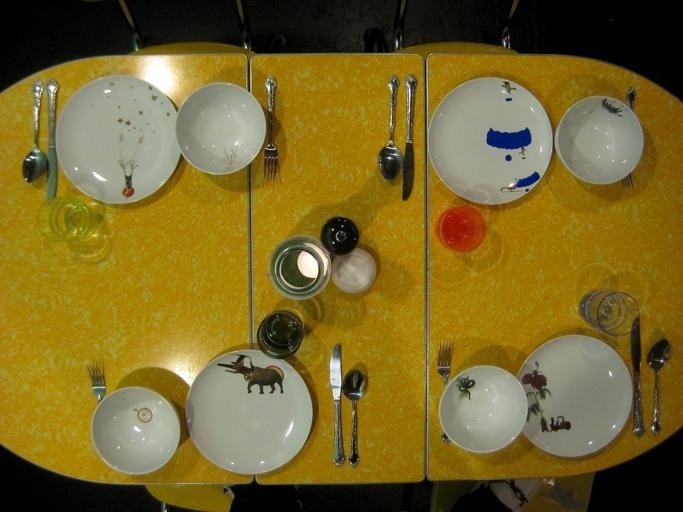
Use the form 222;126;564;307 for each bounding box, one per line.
86;362;107;404
437;339;453;445
262;74;278;185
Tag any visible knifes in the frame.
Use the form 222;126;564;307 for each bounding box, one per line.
329;342;347;467
42;78;60;200
630;316;645;439
401;73;418;201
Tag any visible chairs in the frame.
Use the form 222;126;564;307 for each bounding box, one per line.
119;0;253;55
395;1;521;52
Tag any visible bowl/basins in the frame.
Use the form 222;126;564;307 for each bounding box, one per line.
90;385;182;476
438;364;528;455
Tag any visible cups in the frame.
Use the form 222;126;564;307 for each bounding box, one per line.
580;289;640;338
36;199;99;243
435;207;487;254
274;245;325;294
256;310;304;359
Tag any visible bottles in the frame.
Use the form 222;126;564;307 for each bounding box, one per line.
320;215;376;297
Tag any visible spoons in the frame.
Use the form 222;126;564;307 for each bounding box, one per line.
22;78;49;191
646;338;671;436
342;367;367;467
377;73;404;184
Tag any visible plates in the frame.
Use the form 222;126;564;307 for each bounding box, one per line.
185;349;315;476
268;235;331;301
426;76;644;205
55;73;266;206
516;335;633;459
